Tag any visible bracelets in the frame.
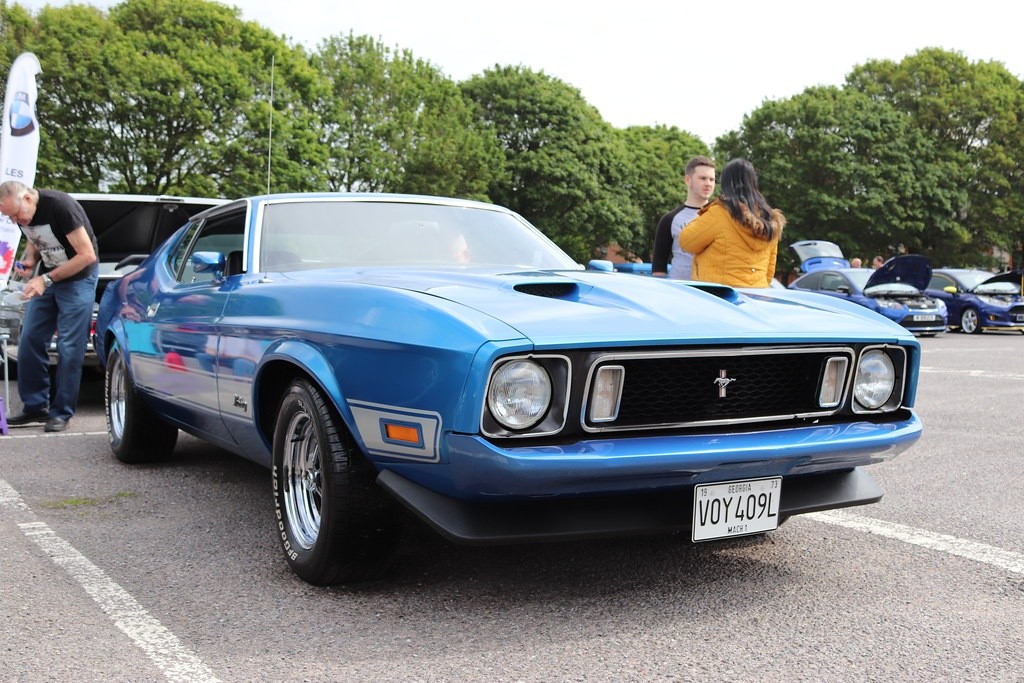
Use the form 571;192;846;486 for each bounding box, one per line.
46;272;54;282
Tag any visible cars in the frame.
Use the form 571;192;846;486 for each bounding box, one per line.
928;268;1024;334
785;240;948;338
94;191;922;587
0;193;235;396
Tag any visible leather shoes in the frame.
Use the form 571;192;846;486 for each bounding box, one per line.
45;417;70;432
6;402;50;425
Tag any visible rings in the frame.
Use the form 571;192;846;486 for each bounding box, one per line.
29;294;33;297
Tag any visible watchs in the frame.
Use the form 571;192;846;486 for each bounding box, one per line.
42;274;53;288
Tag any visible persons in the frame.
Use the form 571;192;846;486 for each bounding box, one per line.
850;258;862;268
651;157;716;279
430;229;472;265
0;182;100;431
679;157;788;288
774;272;783;284
788;263;801;285
873;255;884;270
589;247;618;272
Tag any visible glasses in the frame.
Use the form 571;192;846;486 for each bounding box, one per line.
6;199;22;224
